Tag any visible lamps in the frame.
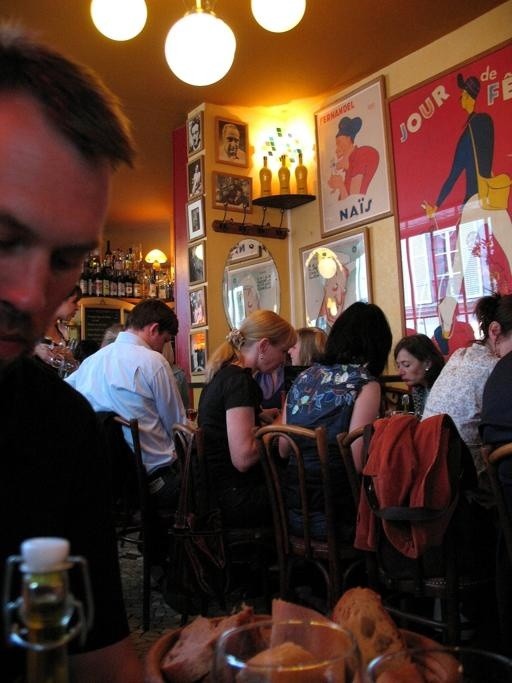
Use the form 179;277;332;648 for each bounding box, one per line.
145;248;169;272
90;1;307;86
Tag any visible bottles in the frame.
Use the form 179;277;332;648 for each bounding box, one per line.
260;156;272;196
79;239;175;299
295;152;308;195
278;155;291;195
20;538;71;679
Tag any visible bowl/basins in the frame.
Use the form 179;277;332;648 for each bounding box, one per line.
144;611;458;682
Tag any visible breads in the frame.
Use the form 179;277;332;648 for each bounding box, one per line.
155;585;426;682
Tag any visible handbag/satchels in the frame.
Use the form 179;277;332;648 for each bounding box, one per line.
161;528;230;614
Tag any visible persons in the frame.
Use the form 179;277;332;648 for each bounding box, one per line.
218;119;245;166
187;117;205;153
38;285;83;377
197;294;512;547
2;33;145;681
189;163;202;194
65;298;188;521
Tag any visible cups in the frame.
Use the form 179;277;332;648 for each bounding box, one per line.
217;619;366;682
